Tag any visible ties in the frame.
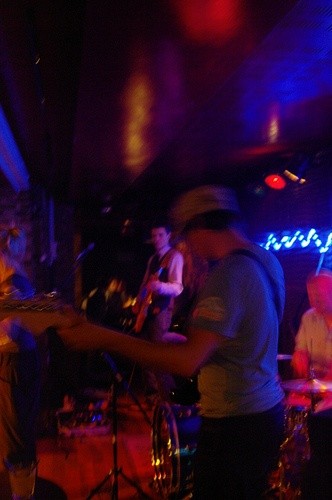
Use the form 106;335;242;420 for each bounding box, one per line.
150;255;159;273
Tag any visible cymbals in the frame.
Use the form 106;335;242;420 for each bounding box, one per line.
280;377;331;396
276;353;295;361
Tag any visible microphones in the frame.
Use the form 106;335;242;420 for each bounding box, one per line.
75;243;95;261
100;351;123;382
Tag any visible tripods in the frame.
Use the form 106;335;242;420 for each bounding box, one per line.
86;378;151;500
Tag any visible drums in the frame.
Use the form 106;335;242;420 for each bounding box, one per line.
147;400;201;500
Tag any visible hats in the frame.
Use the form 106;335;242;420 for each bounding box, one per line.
168;186;241;249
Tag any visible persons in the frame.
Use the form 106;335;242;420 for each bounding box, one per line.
58;186;288;500
130;219;184;343
0;208;36;480
282;269;332;412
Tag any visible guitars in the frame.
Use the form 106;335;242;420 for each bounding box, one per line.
1;293;202;408
124;252;171;328
